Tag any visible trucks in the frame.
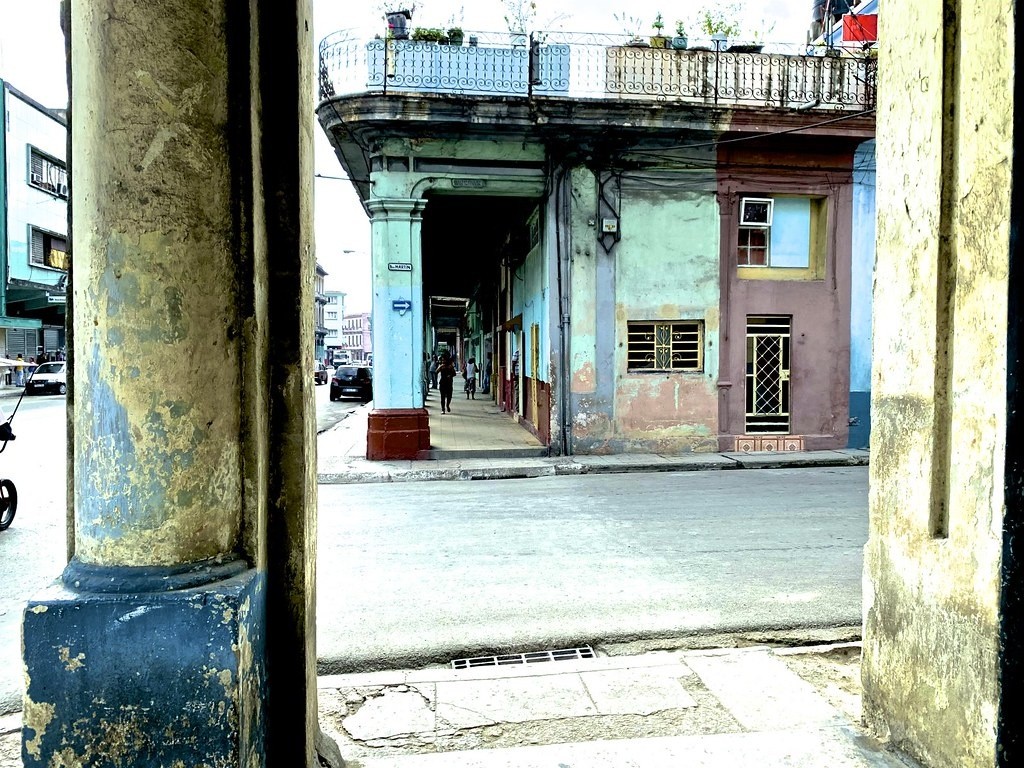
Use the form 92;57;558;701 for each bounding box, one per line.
332;349;353;370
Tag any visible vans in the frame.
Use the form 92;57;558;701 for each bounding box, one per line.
24;360;67;395
365;353;373;367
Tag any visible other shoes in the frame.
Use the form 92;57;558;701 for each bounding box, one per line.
466;397;469;399
472;397;475;400
441;410;445;414
446;404;451;412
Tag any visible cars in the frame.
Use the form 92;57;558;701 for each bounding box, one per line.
352;360;362;367
314;360;328;385
330;365;373;403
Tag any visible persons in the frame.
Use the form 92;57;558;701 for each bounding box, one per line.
464;357;479;400
481;352;492;395
436;354;456;413
5;352;47;387
511;350;521;413
430;355;440;390
